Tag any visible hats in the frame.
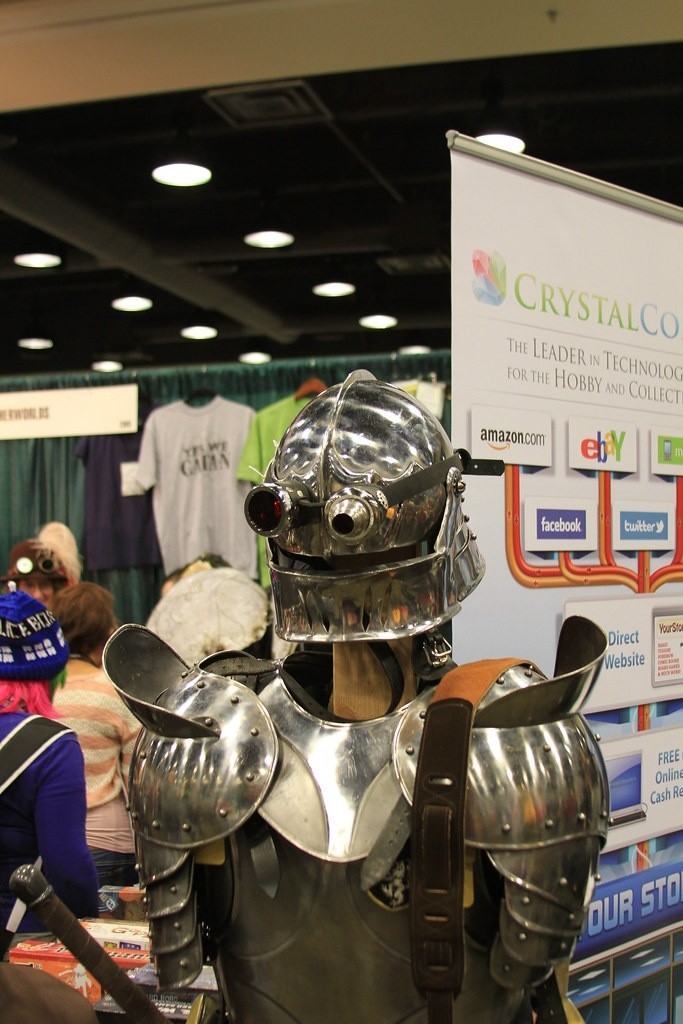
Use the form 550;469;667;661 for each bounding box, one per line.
0;538;69;585
0;590;69;681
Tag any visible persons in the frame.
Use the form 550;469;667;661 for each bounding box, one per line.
2;538;240;919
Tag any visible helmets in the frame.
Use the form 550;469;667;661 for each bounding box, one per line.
242;369;506;646
